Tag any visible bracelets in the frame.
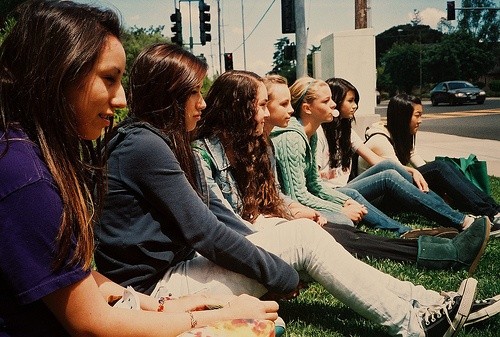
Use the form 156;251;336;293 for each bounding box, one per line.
157;297;164;312
186;311;197;328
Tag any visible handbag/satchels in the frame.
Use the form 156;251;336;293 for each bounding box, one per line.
436;153;493;198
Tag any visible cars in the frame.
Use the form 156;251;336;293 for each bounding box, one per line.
429;80;486;107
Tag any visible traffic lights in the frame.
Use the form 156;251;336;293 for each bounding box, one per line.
169;8;182;46
199;2;211;44
224;52;234;71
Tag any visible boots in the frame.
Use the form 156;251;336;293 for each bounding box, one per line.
415;216;490;274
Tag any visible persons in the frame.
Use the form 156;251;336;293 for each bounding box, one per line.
0;0;279;337
187;70;490;273
260;75;500;241
87;43;499;337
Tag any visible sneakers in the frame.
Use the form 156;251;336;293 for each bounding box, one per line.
420;277;480;337
463;295;500;326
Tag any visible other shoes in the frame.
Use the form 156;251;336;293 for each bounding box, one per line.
463;214;494;231
401;227;459;239
493;214;500;225
